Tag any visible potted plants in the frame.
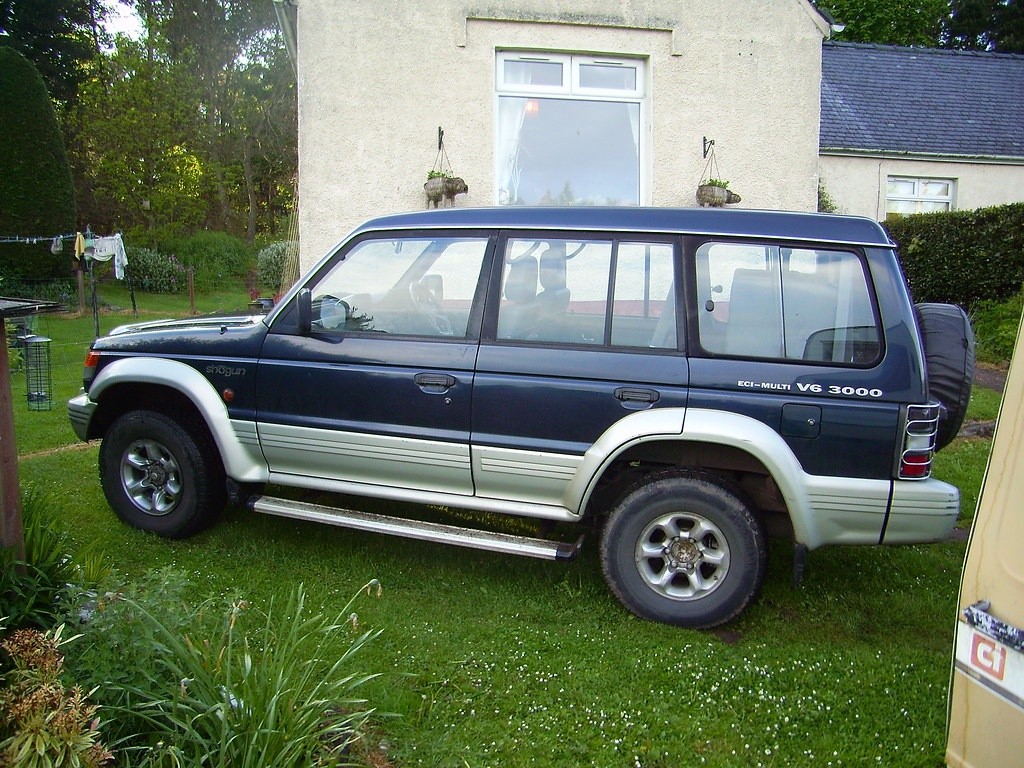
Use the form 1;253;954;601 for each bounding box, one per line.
696;179;742;208
425;170;467;209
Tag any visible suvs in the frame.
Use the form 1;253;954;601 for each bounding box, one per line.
66;205;975;628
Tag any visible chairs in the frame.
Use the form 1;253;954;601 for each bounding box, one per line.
498;248;570;338
